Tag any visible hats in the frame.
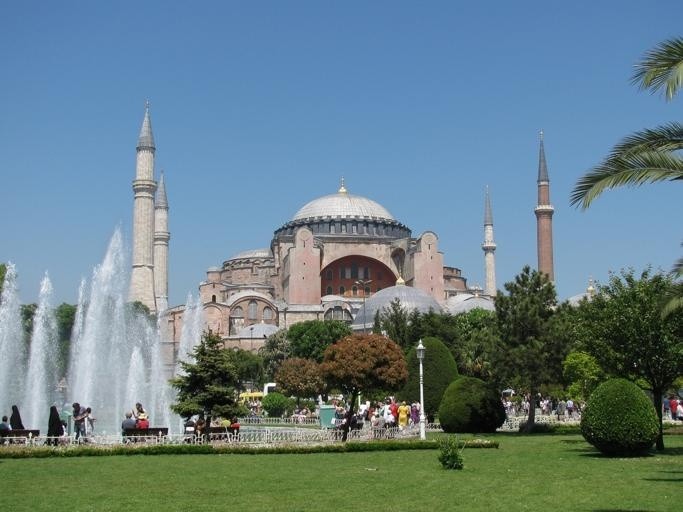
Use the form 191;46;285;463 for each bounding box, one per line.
138;413;148;420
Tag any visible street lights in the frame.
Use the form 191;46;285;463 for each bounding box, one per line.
414;338;426;440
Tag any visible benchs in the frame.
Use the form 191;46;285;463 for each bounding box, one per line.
207;426;238;440
122;426;168;442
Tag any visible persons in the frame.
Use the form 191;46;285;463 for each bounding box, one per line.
2;401;150;446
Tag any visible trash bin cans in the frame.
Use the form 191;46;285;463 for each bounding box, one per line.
319;405;336;428
